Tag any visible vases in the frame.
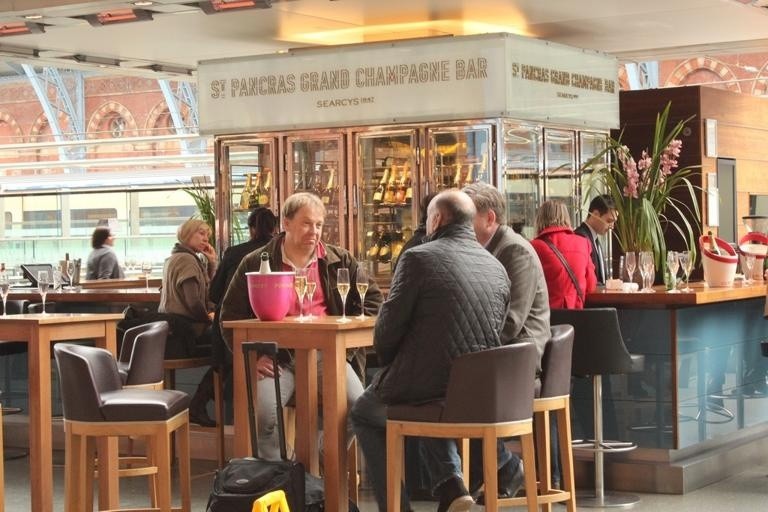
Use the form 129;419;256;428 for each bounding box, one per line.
623;254;656;290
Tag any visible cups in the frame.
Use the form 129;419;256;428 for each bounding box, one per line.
53;266;62;293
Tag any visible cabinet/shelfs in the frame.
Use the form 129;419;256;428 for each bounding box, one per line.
579;131;614;280
346;115;496;290
497;118;578;244
214;129;346;261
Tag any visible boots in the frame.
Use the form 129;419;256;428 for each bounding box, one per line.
186;392;215;426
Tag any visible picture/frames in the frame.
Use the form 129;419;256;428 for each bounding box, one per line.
716;159;738;249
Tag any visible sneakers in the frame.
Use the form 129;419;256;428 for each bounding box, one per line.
436;479;473;511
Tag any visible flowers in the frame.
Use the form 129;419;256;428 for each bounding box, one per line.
550;98;722;287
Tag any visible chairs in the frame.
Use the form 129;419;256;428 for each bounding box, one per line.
533;323;577;512
550;307;647;509
384;342;539;512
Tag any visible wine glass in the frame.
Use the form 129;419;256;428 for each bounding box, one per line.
355;268;370;319
141;260;154;292
0;282;9;317
745;253;756;284
294;267;317;320
337;268;351;322
66;260;77;293
626;249;693;293
37;271;49;314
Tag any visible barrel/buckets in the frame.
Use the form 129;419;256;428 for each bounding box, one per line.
699;235;739;289
736;231;768;282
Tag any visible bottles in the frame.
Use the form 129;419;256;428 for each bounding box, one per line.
312;169;340;206
66;253;69;261
452;151;487;189
707;232;721;255
259;252;272;273
371;165;413;205
367;230;393;262
239;171;271;209
763;249;768;282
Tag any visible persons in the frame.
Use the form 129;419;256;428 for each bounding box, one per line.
526;200;610;495
218;192;384;476
573;196;619;440
394;192;443;274
457;185;551;506
86;228;125;280
158;220;233;427
208;208;277;425
351;189;511;512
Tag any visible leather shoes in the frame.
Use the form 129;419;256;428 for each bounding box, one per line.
477;455;528;505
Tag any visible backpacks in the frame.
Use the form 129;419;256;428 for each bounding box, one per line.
210;454;305;511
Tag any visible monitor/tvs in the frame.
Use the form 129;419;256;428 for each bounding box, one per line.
20;263;68;286
